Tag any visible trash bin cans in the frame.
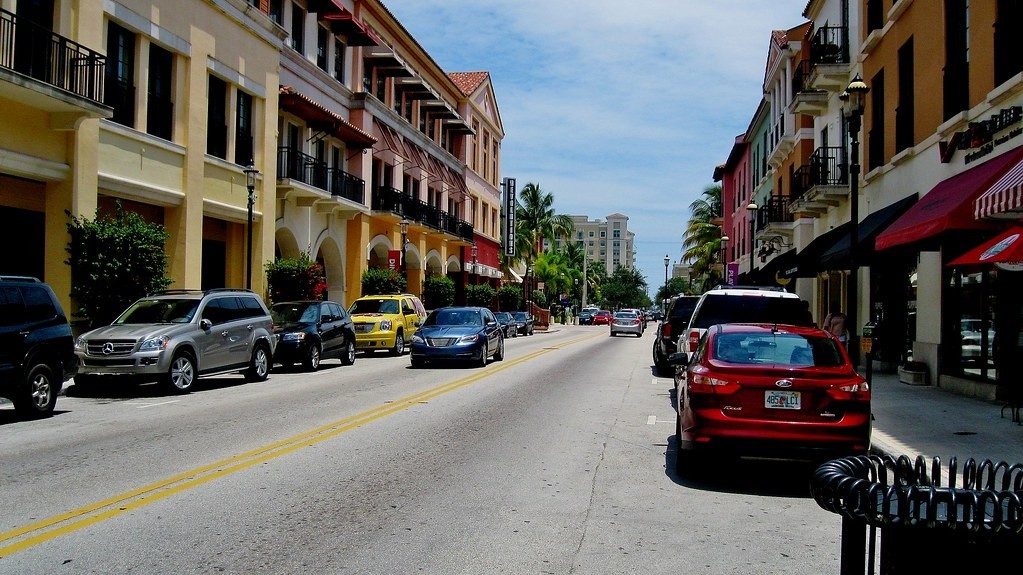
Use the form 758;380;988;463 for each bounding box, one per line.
810;455;1023;575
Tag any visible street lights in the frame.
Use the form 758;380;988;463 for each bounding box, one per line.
687;263;694;291
399;215;409;280
529;261;535;317
243;159;260;292
746;197;758;286
575;278;578;316
838;73;871;373
663;254;670;319
561;273;565;325
470;244;478;307
721;232;730;287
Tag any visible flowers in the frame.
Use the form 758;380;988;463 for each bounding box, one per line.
903;359;931;373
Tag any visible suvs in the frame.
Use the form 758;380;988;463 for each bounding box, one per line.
72;288;278;397
0;276;81;421
652;293;703;376
668;285;814;390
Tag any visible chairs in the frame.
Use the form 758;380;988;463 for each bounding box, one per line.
720;348;749;363
789;346;815;367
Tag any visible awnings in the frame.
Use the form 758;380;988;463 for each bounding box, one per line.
305;0;379;47
946;226;1023;265
279;86;378;150
362;30;476;135
972;159;1023;221
876;145;1023;249
500;267;523;282
719;191;920;291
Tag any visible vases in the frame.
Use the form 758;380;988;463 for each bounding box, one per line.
898;368;928;386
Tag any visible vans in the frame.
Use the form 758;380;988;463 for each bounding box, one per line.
662;299;671;316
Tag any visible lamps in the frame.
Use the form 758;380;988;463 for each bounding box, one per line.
758;235;792;257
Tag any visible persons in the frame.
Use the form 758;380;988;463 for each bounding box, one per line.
557;301;579;325
653;308;658;323
800;300;850;353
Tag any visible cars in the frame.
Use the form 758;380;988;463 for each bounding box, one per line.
669;322;874;481
346;294;428;357
492;311;517;339
509;311;534;336
579;306;661;333
960;319;996;361
609;311;644;337
409;306;504;369
270;300;357;373
593;309;612;326
